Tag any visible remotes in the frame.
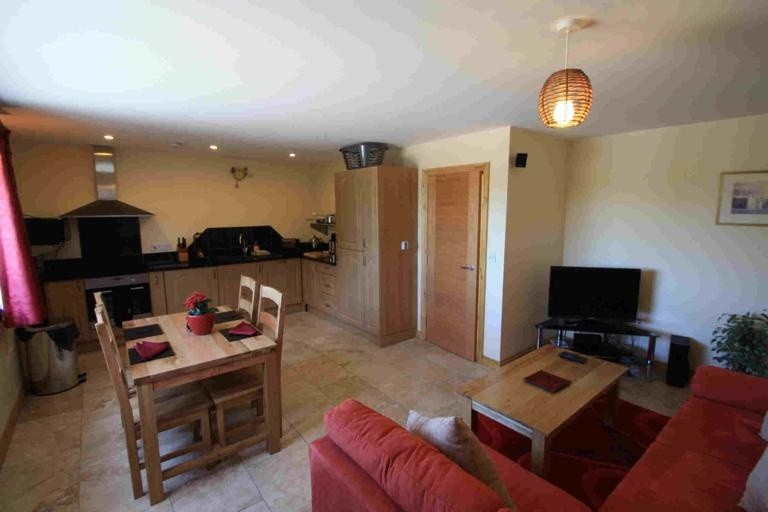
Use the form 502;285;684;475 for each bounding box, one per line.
560;351;587;364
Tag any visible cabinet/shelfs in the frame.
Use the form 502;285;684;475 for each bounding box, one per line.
334;164;418;347
164;257;336;324
44;278;90;344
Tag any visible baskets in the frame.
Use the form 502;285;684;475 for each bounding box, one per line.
338;142;389;170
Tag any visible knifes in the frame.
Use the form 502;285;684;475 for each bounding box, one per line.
177;237;187;249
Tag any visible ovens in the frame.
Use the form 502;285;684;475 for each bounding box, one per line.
83;282;154;335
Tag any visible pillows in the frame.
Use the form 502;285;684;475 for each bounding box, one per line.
758;409;768;441
737;446;768;512
323;397;518;512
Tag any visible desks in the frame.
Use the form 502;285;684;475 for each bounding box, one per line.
534;320;661;382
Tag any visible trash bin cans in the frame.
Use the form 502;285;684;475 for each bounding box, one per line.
15;317;87;396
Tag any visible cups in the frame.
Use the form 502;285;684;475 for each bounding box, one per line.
326;215;336;224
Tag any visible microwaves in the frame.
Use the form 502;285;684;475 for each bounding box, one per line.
25;217;65;246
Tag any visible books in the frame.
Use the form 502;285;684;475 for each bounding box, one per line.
523;369;571;393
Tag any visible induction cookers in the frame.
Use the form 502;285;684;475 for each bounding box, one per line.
80;254;149;289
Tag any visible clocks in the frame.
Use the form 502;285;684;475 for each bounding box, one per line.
231;167;248;188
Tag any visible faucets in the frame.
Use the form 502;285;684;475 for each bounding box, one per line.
239;234;247;256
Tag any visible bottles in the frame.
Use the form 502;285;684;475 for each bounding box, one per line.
241;231;249;257
311;234;319;250
252;240;260;256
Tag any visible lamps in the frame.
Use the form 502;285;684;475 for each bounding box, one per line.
537;18;592;127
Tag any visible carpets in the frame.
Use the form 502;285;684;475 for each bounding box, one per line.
473;394;672;512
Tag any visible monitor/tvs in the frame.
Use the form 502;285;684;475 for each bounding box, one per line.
548;265;641;322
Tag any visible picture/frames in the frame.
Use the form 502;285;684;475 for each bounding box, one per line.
716;171;768;227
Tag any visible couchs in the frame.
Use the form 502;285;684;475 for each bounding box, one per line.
308;366;768;512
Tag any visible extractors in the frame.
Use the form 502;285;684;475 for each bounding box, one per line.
60;146;156;221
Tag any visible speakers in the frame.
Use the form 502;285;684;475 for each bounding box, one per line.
666;334;690;388
